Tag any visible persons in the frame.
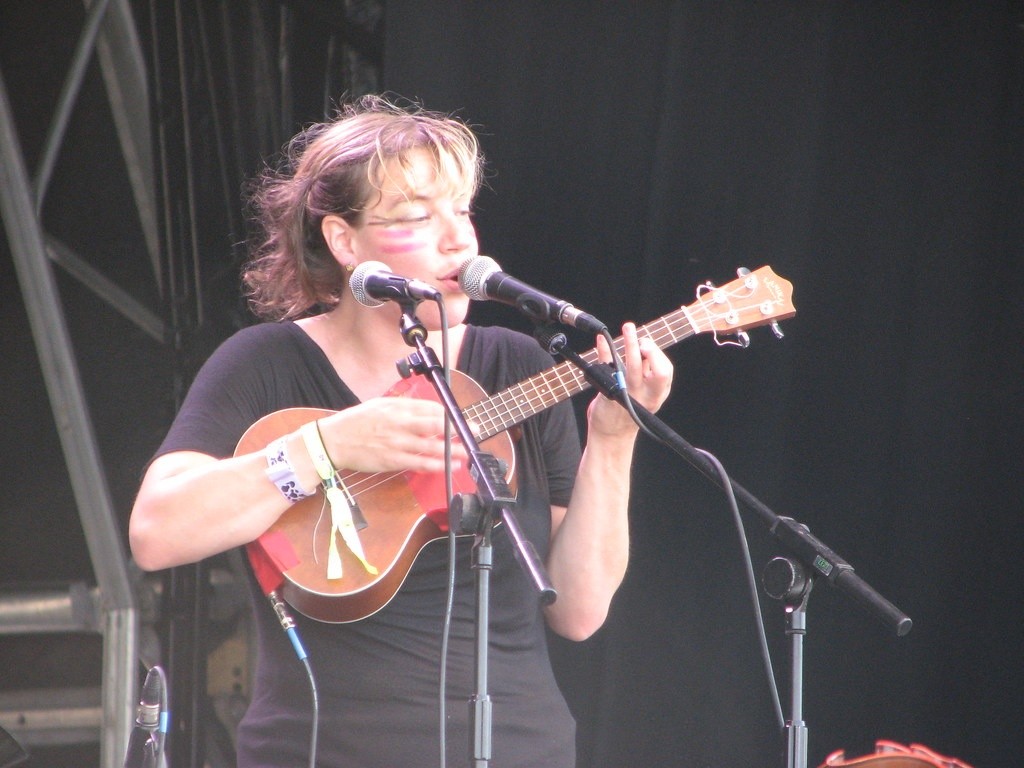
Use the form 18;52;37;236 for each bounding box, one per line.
128;107;676;768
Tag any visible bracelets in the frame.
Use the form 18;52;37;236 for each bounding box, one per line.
264;432;316;504
299;416;380;580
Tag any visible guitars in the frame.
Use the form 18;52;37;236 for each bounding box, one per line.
230;261;799;628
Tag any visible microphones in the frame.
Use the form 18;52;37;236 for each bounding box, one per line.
458;255;608;336
349;260;442;308
123;668;166;768
